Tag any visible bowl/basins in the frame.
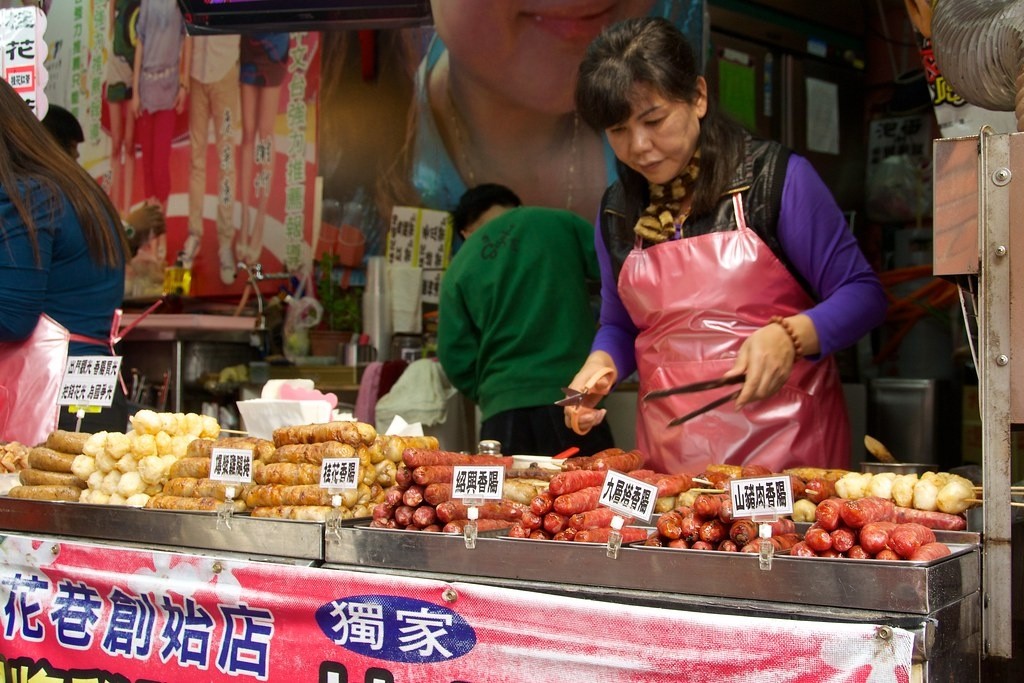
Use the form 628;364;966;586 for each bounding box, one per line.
513;454;568;471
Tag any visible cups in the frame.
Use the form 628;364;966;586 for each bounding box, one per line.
361;255;393;358
861;462;937;477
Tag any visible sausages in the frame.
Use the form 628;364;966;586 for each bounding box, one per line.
370;447;969;567
145;422;439;524
8;429;92;502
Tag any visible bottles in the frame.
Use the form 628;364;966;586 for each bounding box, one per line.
163;250;191;295
477;439;502;459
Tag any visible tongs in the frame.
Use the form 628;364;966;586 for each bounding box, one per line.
642;372;752;427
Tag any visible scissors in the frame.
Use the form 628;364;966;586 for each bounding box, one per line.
554;366;617;437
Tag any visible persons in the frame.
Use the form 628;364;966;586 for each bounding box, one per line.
0;77;165;448
407;0;656;254
563;14;888;477
436;183;616;458
105;0;291;300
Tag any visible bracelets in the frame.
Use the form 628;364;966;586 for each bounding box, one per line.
770;315;804;361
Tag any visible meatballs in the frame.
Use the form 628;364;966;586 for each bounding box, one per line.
70;408;221;508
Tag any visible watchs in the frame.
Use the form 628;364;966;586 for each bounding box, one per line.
122;220;137;243
